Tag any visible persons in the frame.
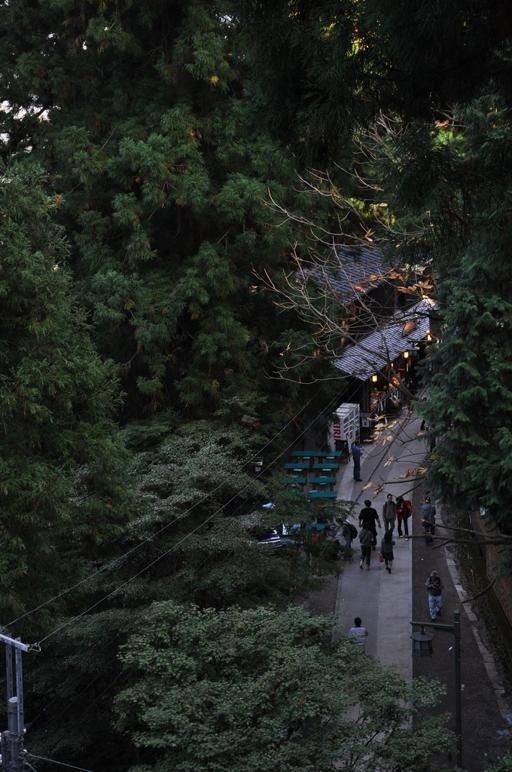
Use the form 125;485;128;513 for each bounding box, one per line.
395;495;412;542
300;515;356;572
350;438;364;483
349;616;370;658
420;497;437;547
358;522;375;572
382;493;397;546
379;528;396;575
425;569;446;625
358;499;381;551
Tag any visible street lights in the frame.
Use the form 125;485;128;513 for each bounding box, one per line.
408;608;465;772
